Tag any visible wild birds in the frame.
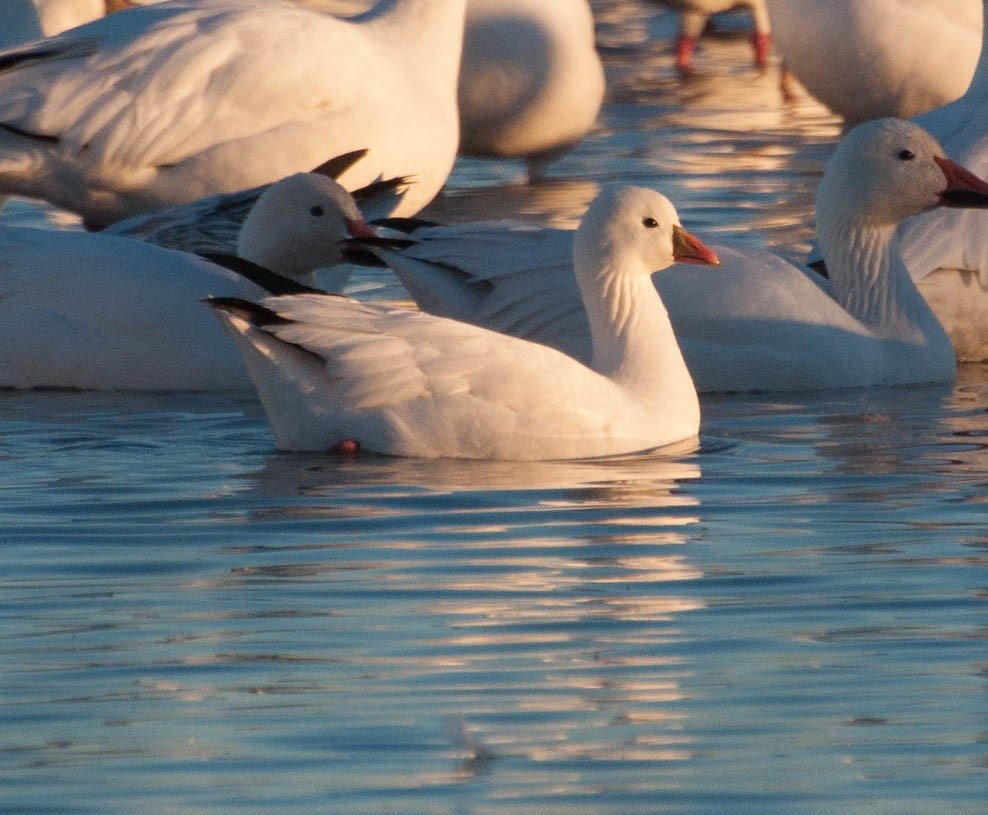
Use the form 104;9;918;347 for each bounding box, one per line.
2;176;392;392
342;116;988;392
197;187;723;458
2;0;988;367
